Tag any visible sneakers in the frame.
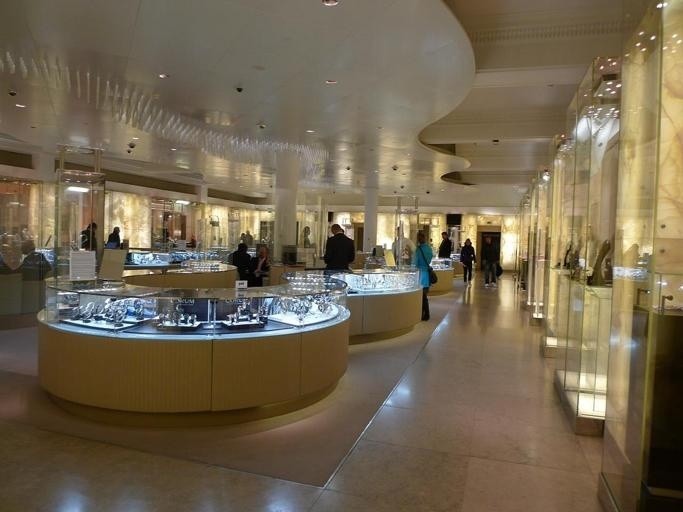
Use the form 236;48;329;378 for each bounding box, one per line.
464;281;472;287
485;282;497;287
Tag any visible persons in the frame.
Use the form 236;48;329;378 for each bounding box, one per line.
16;238;52;281
80;223;97;251
437;231;452;266
106;226;121;248
239;229;253;245
247;246;271;287
231;242;251;283
480;236;499;287
322;223;356;290
457;285;474;330
185;235;197;248
459;238;477;285
390;225;415;266
414;229;434;322
303;226;311;249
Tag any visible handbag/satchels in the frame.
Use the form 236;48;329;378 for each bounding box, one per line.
495;263;502;276
428;267;437;283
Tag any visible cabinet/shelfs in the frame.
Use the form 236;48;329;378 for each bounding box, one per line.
36;252;464;412
